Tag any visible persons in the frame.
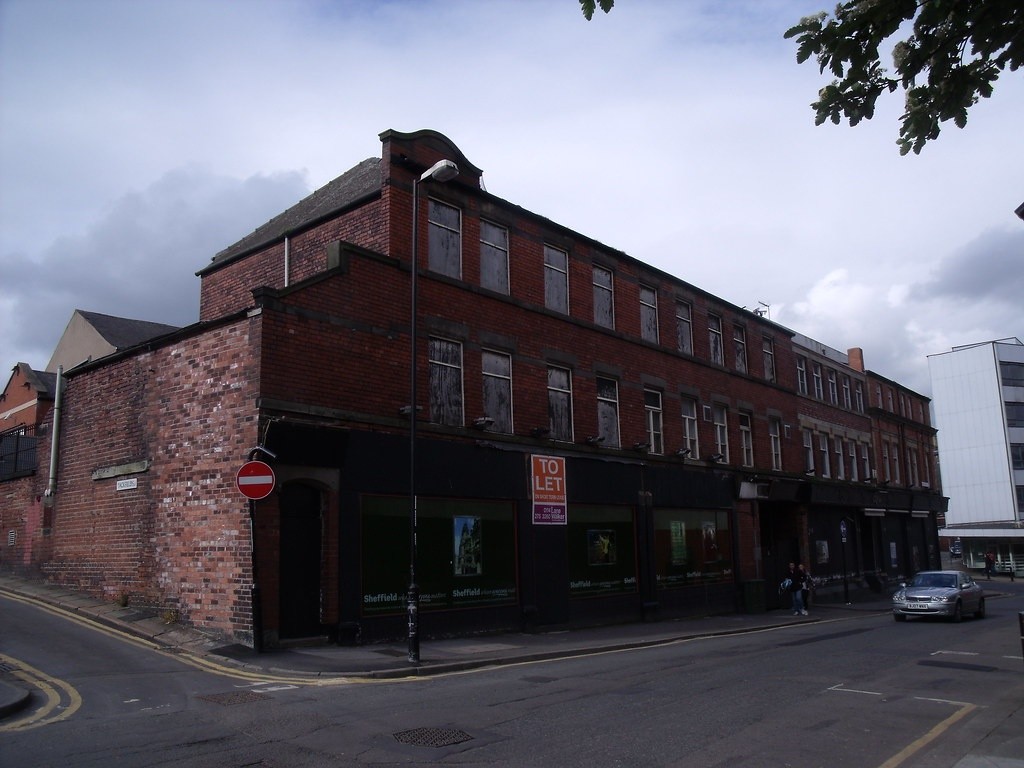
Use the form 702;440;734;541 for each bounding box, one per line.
783;560;811;616
985;549;998;577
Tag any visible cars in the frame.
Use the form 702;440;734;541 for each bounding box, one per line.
892;568;985;623
948;541;962;558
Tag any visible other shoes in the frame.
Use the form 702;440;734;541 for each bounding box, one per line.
802;609;805;615
793;612;798;616
805;611;808;616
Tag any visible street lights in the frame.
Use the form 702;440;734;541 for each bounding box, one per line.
404;160;459;660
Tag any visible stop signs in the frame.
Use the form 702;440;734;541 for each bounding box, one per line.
236;461;275;500
